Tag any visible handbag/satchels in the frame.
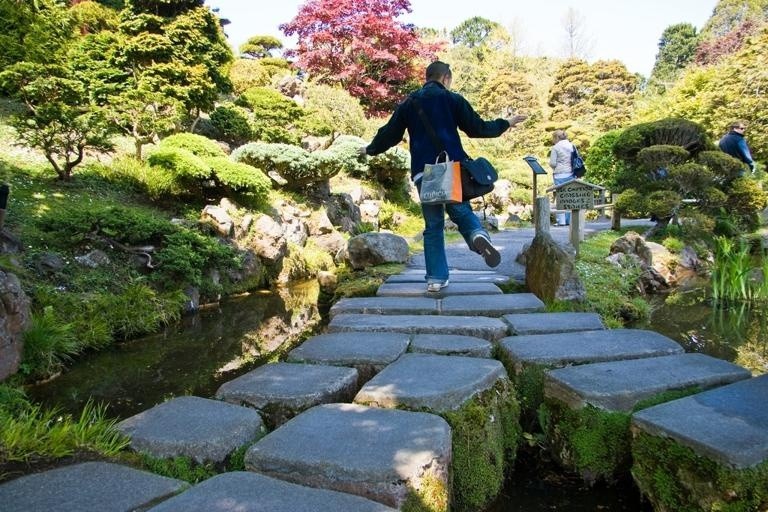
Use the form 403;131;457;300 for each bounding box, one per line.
461;157;498;202
573;145;587;178
420;151;463;204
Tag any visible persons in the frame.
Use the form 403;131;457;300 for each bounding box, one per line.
550;130;574;225
720;121;757;177
356;60;527;292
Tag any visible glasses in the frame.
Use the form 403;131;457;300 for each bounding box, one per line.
736;128;745;131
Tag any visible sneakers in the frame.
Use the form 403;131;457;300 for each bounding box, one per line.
473;234;502;268
427;280;449;292
